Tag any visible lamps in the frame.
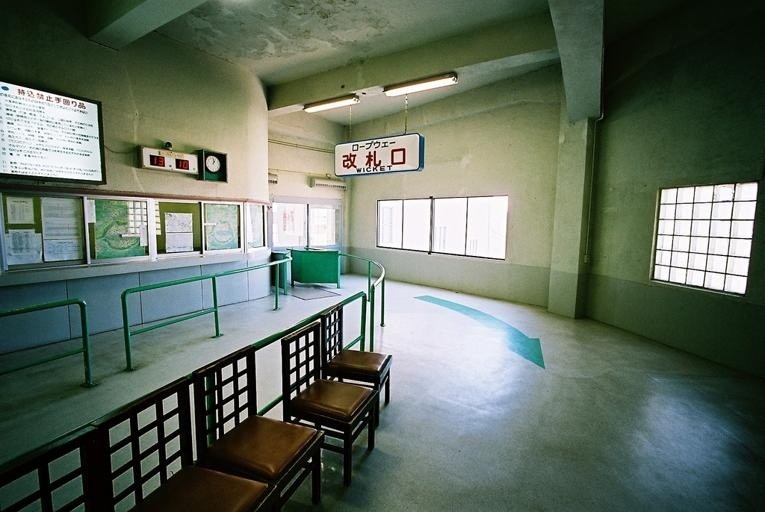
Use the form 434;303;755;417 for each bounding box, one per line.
300;93;362;115
379;70;460;98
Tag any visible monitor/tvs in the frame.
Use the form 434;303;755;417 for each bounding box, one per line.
0;79;107;185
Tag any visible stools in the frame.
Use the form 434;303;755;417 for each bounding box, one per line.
281;380;380;487
123;464;283;512
199;413;327;512
321;349;392;426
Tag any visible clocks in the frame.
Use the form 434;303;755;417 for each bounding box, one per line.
205;154;223;175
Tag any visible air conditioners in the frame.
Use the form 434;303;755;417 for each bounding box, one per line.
309;175;347;193
